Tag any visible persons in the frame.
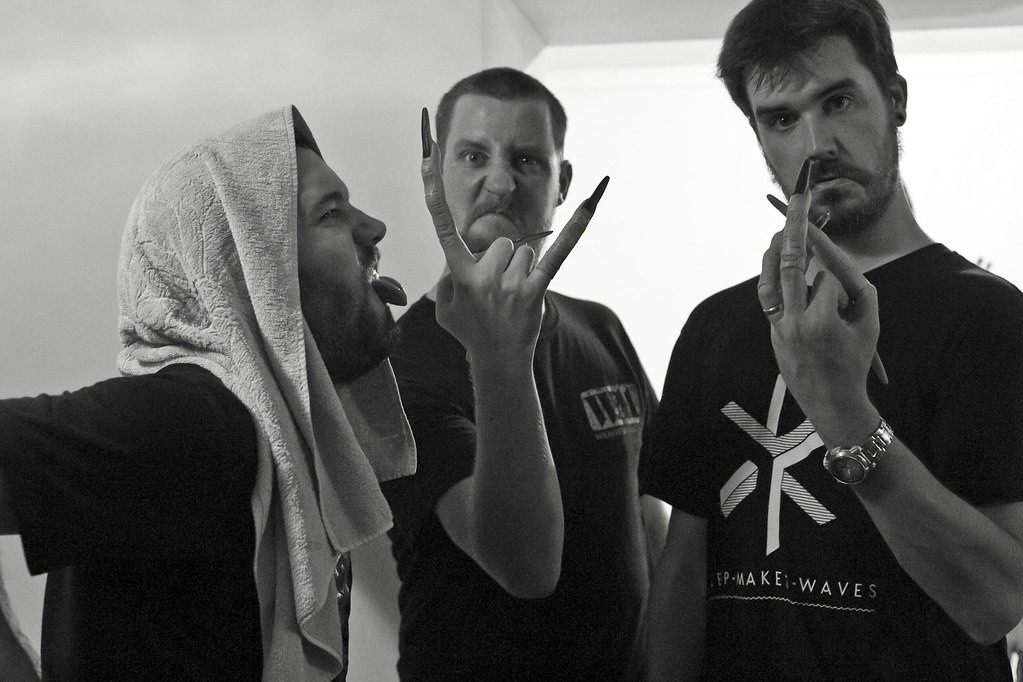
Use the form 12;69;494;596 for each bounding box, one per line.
639;0;1023;682
0;106;417;682
378;66;671;682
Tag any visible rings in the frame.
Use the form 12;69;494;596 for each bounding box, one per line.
763;302;783;315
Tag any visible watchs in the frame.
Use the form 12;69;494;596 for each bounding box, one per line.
822;416;893;485
